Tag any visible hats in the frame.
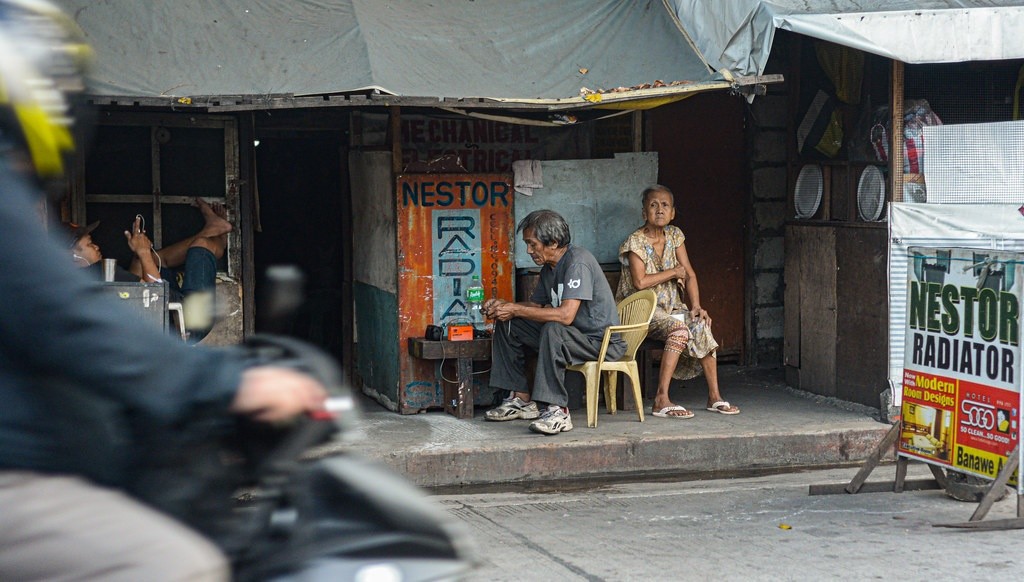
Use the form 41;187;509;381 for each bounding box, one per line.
61;220;100;249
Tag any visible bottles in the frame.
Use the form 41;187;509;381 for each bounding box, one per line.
464;275;486;329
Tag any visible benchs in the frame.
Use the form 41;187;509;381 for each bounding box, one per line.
409;337;665;418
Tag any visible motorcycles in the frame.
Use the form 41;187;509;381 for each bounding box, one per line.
102;328;481;582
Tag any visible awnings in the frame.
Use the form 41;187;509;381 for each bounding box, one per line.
36;2;729;127
676;2;1023;99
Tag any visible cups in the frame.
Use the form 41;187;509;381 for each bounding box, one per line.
100;258;117;282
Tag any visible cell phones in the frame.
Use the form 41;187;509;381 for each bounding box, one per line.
132;218;141;233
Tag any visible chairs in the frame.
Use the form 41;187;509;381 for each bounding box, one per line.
566;289;657;427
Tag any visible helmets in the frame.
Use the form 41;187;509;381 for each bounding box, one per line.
0;0;94;180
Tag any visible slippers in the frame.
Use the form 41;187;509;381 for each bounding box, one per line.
707;401;740;415
651;404;694;419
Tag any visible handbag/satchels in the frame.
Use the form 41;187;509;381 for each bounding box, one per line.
796;87;854;163
870;108;944;176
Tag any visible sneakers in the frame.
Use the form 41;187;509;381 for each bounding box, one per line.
484;390;541;422
529;404;573;435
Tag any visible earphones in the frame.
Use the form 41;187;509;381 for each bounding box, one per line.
73;254;77;258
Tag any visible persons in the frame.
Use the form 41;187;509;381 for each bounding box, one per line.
615;185;739;419
479;209;628;435
57;197;233;345
0;0;329;582
